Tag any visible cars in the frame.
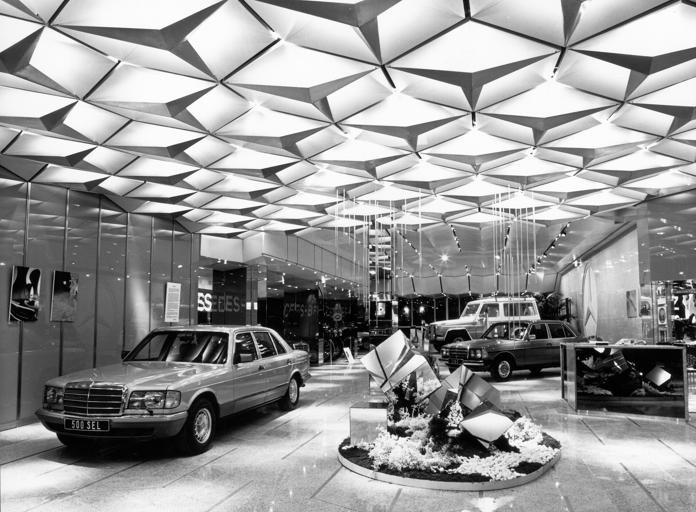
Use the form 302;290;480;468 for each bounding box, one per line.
33;324;310;454
437;319;584;382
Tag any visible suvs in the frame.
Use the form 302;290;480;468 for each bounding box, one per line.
428;298;542;354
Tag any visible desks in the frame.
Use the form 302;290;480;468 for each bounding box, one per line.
560;342;688;420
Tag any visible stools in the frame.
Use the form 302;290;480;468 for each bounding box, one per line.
349;402;388;446
